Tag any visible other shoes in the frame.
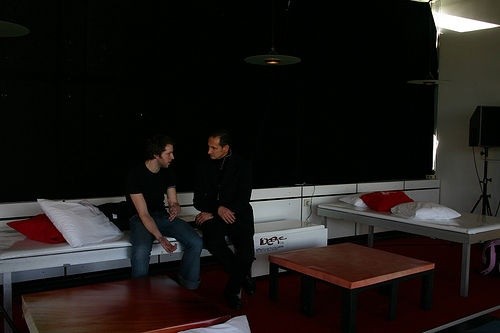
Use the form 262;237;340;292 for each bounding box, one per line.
243;273;257;296
226;286;243;312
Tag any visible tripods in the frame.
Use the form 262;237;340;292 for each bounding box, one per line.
470;147;492;216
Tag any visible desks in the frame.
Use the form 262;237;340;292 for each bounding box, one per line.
267;243;435;333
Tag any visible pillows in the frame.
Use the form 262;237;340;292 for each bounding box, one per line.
338;191;461;222
6;198;132;245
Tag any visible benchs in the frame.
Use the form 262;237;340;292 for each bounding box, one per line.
316;202;500;297
0;216;318;333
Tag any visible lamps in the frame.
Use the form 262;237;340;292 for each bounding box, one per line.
408;0;453;86
244;0;302;64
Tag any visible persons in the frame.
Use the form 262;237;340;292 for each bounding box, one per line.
122;132;203;280
192;130;255;305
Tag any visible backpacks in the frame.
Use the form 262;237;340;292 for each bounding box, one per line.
479;239;500;279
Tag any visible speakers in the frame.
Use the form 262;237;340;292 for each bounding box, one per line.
469;105;500;147
